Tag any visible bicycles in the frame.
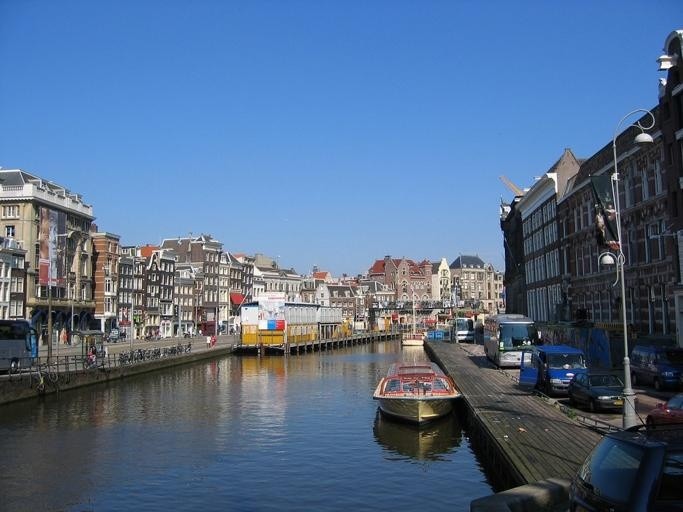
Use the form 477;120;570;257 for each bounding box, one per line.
118;344;191;366
82;356;98;369
32;360;59;392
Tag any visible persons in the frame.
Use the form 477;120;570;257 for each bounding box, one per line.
205;334;211;349
89;343;96;364
210;333;217;348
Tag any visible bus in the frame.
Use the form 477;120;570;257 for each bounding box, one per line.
0;319;38;374
484;314;540;367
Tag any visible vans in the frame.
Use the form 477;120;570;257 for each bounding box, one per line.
518;345;588;394
622;344;682;391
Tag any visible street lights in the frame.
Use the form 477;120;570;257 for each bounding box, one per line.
597;109;653;429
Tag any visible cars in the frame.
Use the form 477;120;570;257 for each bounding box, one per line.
646;391;682;437
569;421;682;512
568;370;637;412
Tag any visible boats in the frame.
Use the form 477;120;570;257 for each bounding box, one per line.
372;362;461;423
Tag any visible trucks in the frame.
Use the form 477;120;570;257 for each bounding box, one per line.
452;318;474;344
